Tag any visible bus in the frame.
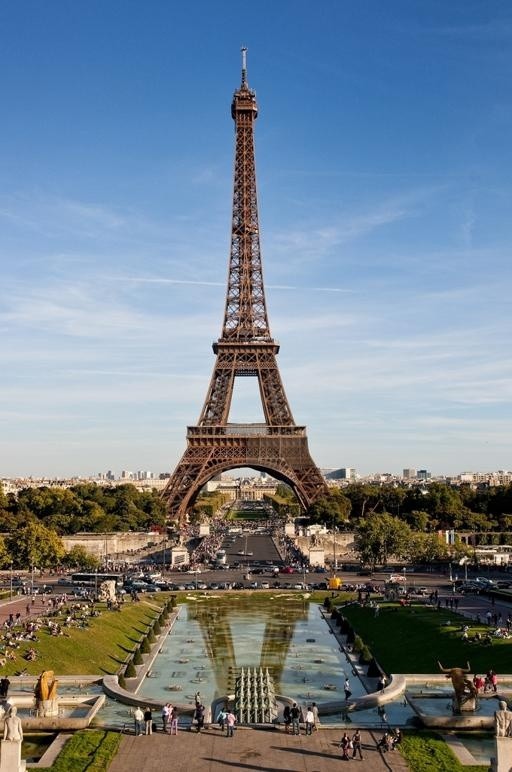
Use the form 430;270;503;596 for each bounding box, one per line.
216;550;227;564
71;573;124;587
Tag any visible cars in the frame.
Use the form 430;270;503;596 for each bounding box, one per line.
264;565;302;574
341;572;431;595
220;560;240;571
129;572;220;591
57;578;71;585
12;576;54;594
233;581;328;590
454;577;512;595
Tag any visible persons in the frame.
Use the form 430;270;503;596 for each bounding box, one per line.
133;706;153;736
472;670;497;694
283;702;319;736
429;589;459;614
344;678;352;700
366;591;370;602
492;700;511;738
162;702;179;735
341;728;365;761
379;674;388;694
374;602;380;617
0;590;141;665
215;708;236;738
377;728;401;751
460;610;512;647
194;702;205;732
4;706;23;741
